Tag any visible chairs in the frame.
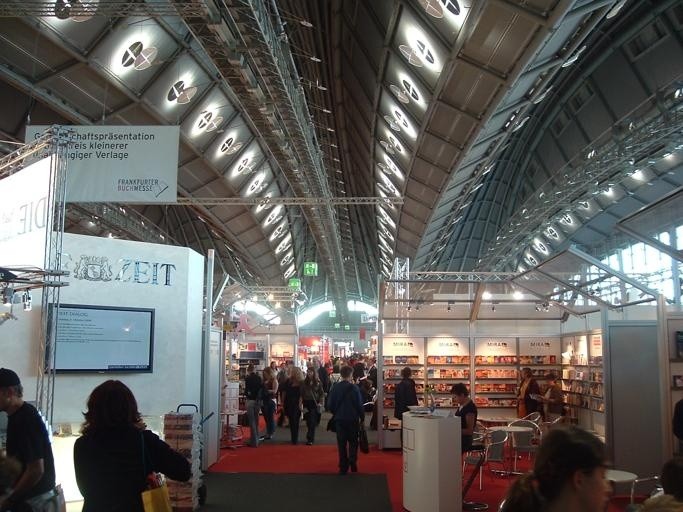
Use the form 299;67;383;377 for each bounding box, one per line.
463;419;488;476
535;414;563;437
474;429;508;489
507;413;540;461
504;419;543;474
628;473;665;506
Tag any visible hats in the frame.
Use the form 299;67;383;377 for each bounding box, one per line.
1;368;20;386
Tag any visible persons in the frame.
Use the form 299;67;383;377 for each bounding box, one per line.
368;363;377;392
449;382;478;452
276;359;294;428
637;459;683;512
331;351;368;379
1;367;61;511
242;362;262;448
271;359;279;377
537;372;564;429
232;353;237;360
260;366;278;440
277;363;286;382
318;363;330;394
298;366;325;446
502;425;614;511
277;367;305;445
306;357;312;369
325;366;365;475
393;366;418;455
514;366;545;421
357;379;377;430
325;359;333;376
73;379;191;512
671;398;683;440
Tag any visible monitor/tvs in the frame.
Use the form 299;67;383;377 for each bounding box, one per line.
45;302;155;377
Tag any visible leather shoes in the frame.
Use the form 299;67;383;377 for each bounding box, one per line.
351;461;356;472
339;469;346;473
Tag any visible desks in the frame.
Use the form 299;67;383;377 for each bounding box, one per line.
605;470;637;505
219;411;249;450
488;426;533;476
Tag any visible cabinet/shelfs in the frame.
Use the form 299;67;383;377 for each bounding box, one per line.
588;364;604;415
519;364;561;396
561;364;589;410
383;363;425;449
428;364;471;408
477;363;519;409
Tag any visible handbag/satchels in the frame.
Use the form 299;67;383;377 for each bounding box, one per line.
326;419;337;432
141;429;172;512
27;484;66;512
317;404;325;413
359;430;370;454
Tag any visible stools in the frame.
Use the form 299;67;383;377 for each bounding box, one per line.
462;442;488;511
